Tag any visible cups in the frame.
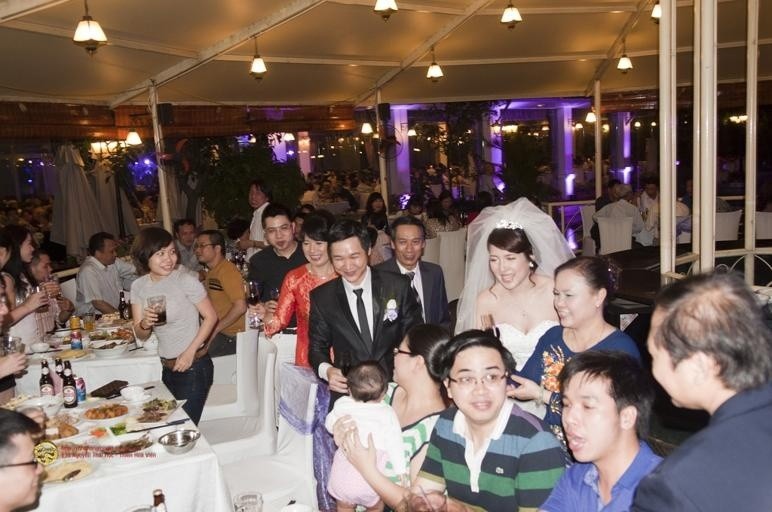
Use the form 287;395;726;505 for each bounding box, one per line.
148;296;166;324
233;491;263;512
45;273;60;298
121;386;144;400
82;312;96;332
143;332;159;351
3;337;22;354
34;286;48;312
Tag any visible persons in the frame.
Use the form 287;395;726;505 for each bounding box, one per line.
628;268;772;511
324;362;406;511
0;194;248;427
333;325;454;512
410;163;770;286
236;165;449;411
0;408;45;512
506;255;642;427
398;328;565;512
456;198;578;371
534;349;665;512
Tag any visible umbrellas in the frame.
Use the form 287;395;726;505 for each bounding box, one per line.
90;154;140;240
50;143;106;256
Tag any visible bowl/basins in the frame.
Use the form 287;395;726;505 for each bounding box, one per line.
16;395;65;419
31;342;50;353
158;429;201;454
54;328;72;338
90;339;129;356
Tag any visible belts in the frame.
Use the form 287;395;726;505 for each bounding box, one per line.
160;346;207;369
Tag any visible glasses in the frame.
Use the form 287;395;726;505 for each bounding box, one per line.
392;347;411;355
200;242;214;248
0;461;36;468
448;371;508;387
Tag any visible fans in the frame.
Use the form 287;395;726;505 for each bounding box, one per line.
371;123;404;159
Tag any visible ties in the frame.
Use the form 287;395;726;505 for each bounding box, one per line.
407;272;422;312
353;288;372;344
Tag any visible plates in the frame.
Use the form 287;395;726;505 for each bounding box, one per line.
77;404;133;423
41;455;106;485
118;394;151;407
38;422;101;444
125;398;188;433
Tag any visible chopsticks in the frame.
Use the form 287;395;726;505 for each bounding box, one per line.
27;349;63;356
46;327;83;334
107;385;154;399
128;418;189;432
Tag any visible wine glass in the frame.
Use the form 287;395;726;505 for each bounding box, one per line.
233;251;241;272
271;287;281;314
244;279;264;328
241;251;248;278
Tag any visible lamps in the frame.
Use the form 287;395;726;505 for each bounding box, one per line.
124;100;144;145
408;121;417;137
374;0;399;25
649;0;661;24
584;106;596;125
361;115;374;136
617;32;633;74
70;0;109;56
426;41;445;83
499;1;524;30
249;35;267;80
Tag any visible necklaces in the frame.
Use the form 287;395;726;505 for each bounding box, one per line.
509;288;537;318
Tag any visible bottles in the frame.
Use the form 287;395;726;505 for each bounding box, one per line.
61;361;78;407
153;489;167;512
119;292;129;321
55;357;64;395
40;359;55;396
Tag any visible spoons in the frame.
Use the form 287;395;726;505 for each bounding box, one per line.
42;469;81;485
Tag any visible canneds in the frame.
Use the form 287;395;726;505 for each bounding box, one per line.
70;330;82;350
70;315;80;330
74;377;86;402
